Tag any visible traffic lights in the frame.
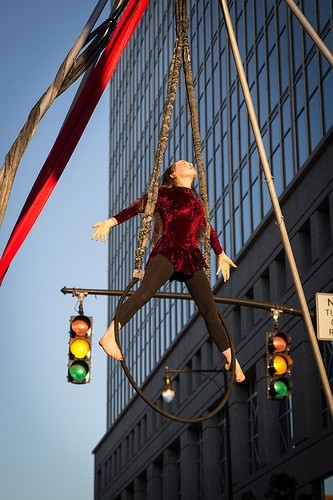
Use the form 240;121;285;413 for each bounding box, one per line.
66;315;93;385
265;331;295;401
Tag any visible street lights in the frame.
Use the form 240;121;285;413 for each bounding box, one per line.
161;367;233;500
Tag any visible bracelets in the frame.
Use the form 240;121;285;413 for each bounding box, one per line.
109;217;117;226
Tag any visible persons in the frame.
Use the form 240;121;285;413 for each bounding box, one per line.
91;159;248;384
